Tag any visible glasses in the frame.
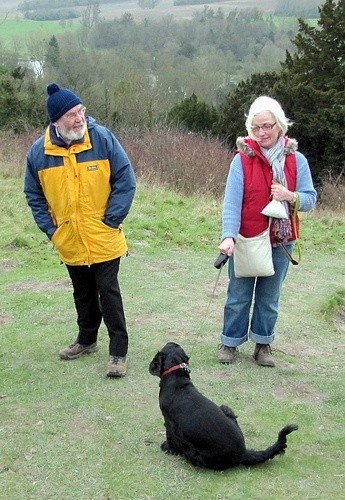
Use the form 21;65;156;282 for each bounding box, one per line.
252;121;277;132
60;107;87;121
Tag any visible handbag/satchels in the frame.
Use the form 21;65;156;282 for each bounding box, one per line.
234;227;275;278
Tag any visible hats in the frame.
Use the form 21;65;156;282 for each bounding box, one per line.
46;84;81;122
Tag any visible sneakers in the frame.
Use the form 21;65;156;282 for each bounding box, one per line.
59;342;98;360
106;356;127;376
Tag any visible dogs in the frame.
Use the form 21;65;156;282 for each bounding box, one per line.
148;342;300;470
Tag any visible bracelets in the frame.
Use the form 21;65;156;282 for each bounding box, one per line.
289;193;296;204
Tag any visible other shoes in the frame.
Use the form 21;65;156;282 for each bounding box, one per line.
218;344;239;362
253;344;275;366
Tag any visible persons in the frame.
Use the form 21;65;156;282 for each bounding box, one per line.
217;95;318;368
24;84;136;378
